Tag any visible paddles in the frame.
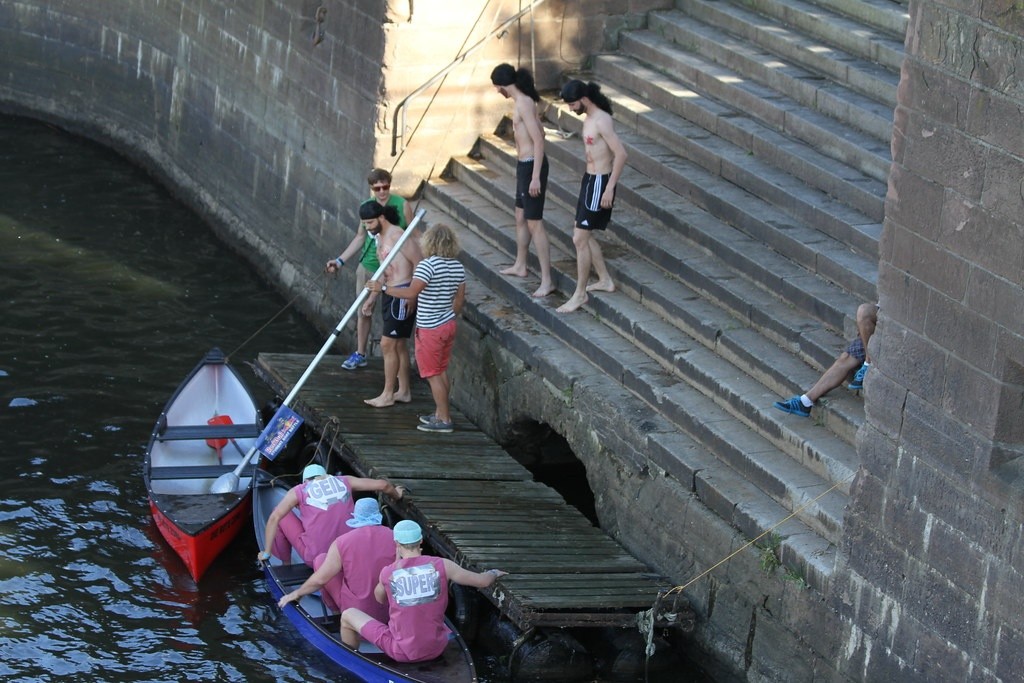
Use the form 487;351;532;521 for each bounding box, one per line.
205;414;252;466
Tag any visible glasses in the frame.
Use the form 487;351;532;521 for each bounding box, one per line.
370;184;390;192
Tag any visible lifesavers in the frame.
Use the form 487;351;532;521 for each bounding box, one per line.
298;440;479;644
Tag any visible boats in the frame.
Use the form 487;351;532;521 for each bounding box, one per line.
143;346;265;583
253;466;477;682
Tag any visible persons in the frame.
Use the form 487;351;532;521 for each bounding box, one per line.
772;301;879;418
258;464;405;569
554;79;627;313
277;497;396;624
358;199;424;407
326;168;419;377
340;520;510;662
364;223;466;433
490;63;556;297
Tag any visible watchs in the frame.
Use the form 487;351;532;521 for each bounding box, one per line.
381;284;387;293
489;570;497;576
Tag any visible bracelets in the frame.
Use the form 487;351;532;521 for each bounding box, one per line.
263;552;270;559
336;257;344;266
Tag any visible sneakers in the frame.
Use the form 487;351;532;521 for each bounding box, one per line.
774;396;812;417
849;365;868;389
417;419;454;432
420;413;451;425
342;351;368;369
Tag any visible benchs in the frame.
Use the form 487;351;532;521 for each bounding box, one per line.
316;614;343;633
273;562;315;586
150;463;255;480
161;424;261;441
